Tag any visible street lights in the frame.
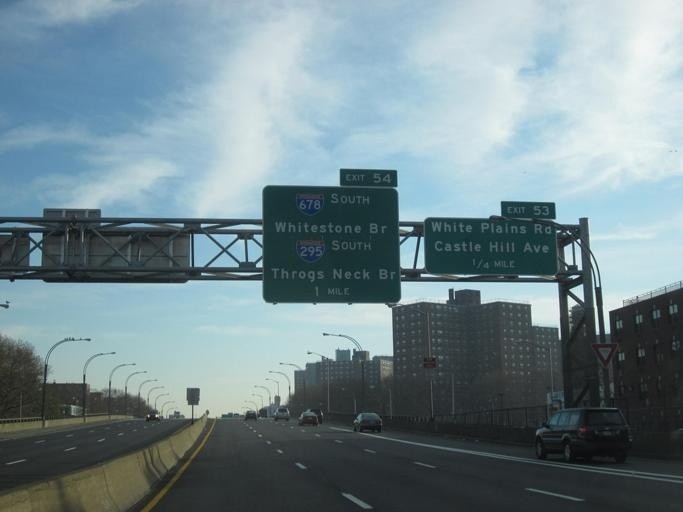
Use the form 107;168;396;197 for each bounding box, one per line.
514;340;554;400
242;332;365;412
41;337;179;427
0;300;10;309
385;302;433;419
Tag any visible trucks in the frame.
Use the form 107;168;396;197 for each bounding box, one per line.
260;409;267;418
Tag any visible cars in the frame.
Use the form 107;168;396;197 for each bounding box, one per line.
534;408;633;463
245;411;256;419
298;408;323;426
275;407;290;421
353;413;382;432
